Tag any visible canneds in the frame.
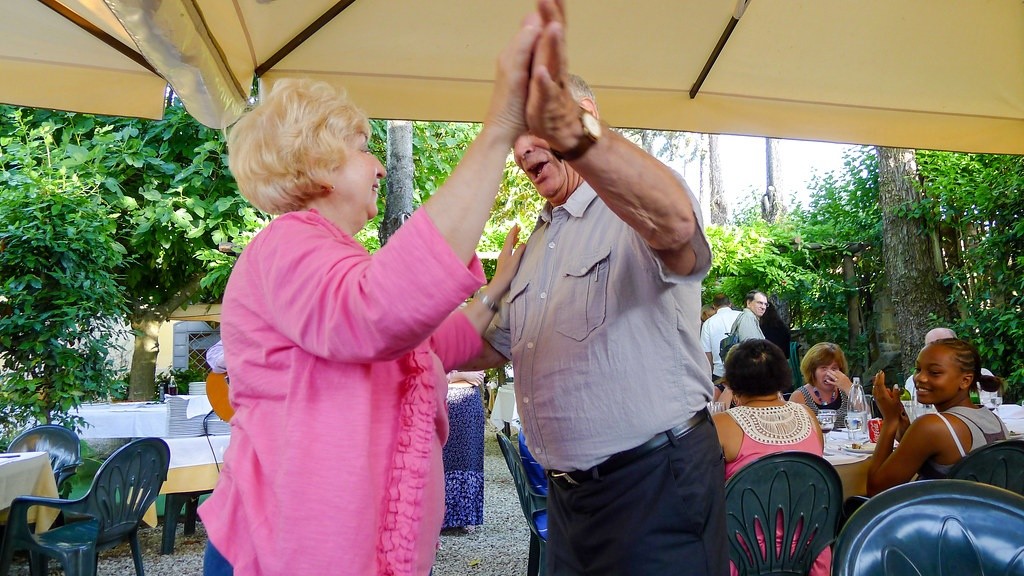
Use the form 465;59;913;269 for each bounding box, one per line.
868;417;883;443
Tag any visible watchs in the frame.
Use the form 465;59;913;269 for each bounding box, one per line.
475;285;499;312
550;107;602;161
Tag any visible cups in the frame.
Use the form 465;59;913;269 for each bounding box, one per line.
904;388;937;424
707;401;726;415
1022;401;1024;409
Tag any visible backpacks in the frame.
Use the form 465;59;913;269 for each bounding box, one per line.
718;312;744;368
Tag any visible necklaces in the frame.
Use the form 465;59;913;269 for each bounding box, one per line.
813;387;835;405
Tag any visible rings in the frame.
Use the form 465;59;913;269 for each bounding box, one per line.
834;378;837;381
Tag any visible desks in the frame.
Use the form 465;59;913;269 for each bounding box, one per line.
122;434;232;553
488;383;523;440
29;401;167;446
821;427;1024;501
0;451;61;535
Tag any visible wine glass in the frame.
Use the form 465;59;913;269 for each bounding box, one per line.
991;392;1003;414
818;409;838;456
844;416;863;447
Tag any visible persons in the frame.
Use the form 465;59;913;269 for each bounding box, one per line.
701;293;743;383
730;290;767;343
867;338;1009;498
197;23;541;576
905;327;958;409
711;338;831;576
789;342;861;429
440;1;726;576
446;370;484;385
206;340;227;374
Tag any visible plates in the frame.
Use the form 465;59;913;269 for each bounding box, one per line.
842;443;877;454
188;382;206;395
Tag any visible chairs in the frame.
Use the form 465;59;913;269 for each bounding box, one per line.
838;437;1024;534
722;451;843;576
494;431;547;576
7;423;80;561
0;437;170;576
829;479;1024;576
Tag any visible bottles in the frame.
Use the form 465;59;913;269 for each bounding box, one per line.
895;373;910;419
159;381;165;401
871;376;882;419
847;377;868;434
970;388;980;406
168;375;179;395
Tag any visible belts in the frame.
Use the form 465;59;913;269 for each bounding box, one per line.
551;405;711;490
